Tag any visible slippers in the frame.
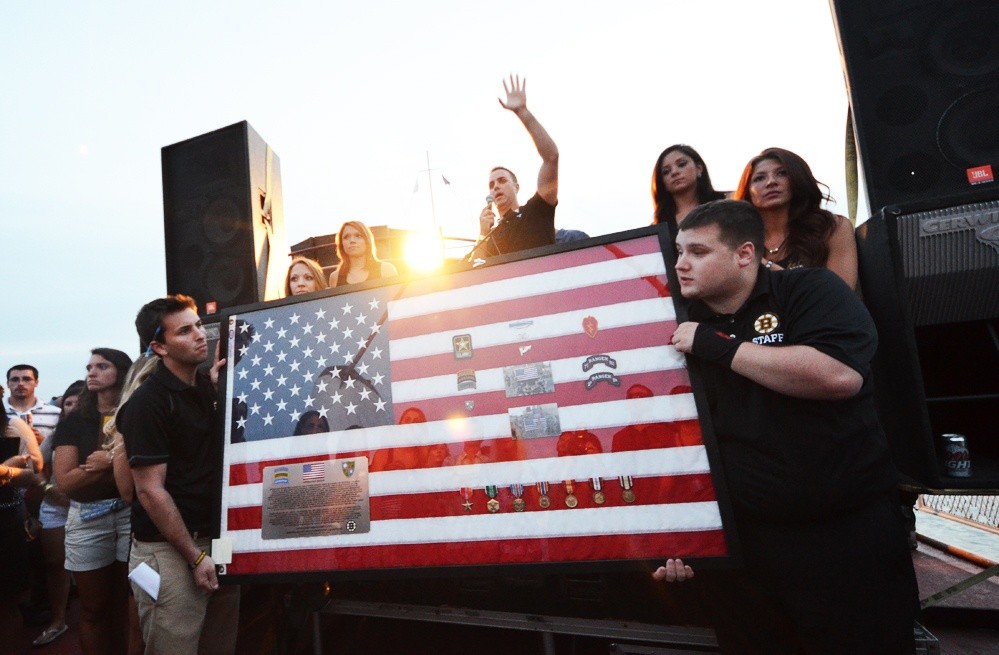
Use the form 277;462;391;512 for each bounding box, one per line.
32;623;69;646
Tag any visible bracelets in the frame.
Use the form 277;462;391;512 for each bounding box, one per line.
764;260;775;271
190;551;208;571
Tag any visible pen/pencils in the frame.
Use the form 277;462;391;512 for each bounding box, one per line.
144;325;160;359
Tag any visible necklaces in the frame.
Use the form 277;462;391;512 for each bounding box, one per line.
761;234;789;253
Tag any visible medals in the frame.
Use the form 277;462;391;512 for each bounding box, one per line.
462;500;474;515
592;490;606;504
487;498;500;513
539;495;549;508
513;498;526;510
566;494;577;508
623;488;634;503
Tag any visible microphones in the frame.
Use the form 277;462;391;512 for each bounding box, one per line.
485;194;494;226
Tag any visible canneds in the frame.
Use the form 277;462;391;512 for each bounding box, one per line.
940;433;972;477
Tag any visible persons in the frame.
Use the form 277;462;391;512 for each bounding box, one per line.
731;147;863;318
285;257;326;297
229;380;714;559
647;143;737;235
639;198;923;655
230;316;254;445
462;73;559;261
0;345;137;654
103;351;161;651
121;293;246;655
327;220;397;295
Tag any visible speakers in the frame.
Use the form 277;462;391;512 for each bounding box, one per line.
854;186;999;327
831;0;999;215
160;119;292;319
